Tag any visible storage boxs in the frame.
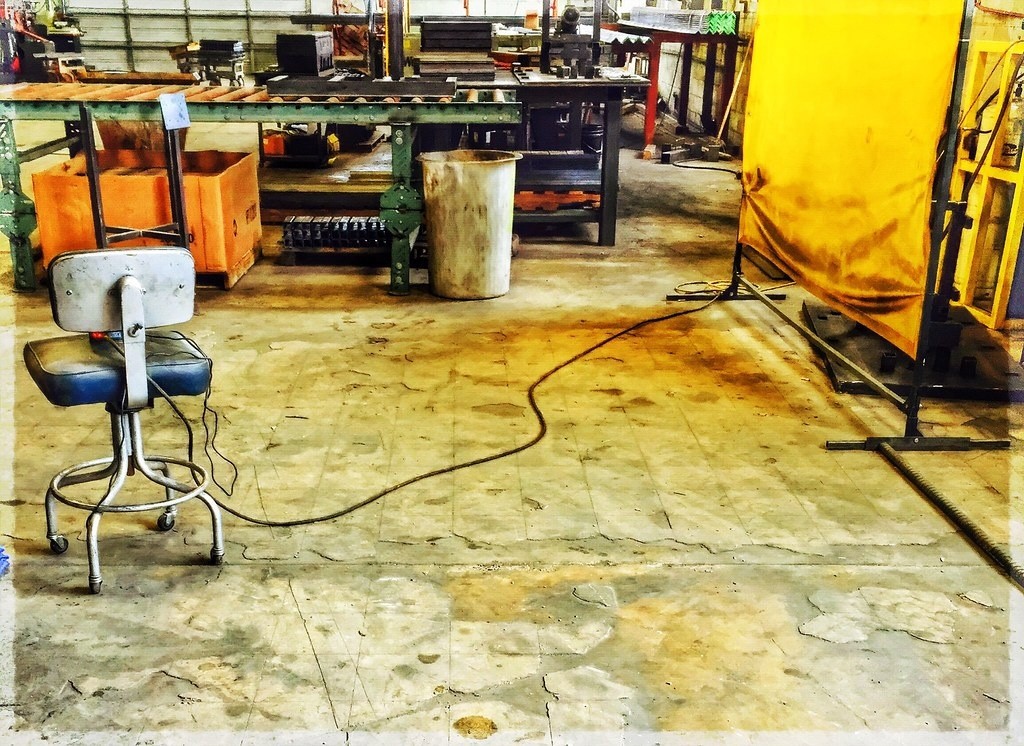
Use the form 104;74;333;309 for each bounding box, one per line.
31;150;261;271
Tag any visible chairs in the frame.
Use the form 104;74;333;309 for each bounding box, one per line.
25;246;222;593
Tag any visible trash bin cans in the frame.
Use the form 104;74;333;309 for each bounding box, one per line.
415;150;524;302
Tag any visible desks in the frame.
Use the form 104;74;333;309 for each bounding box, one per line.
403;66;651;246
601;23;750;149
